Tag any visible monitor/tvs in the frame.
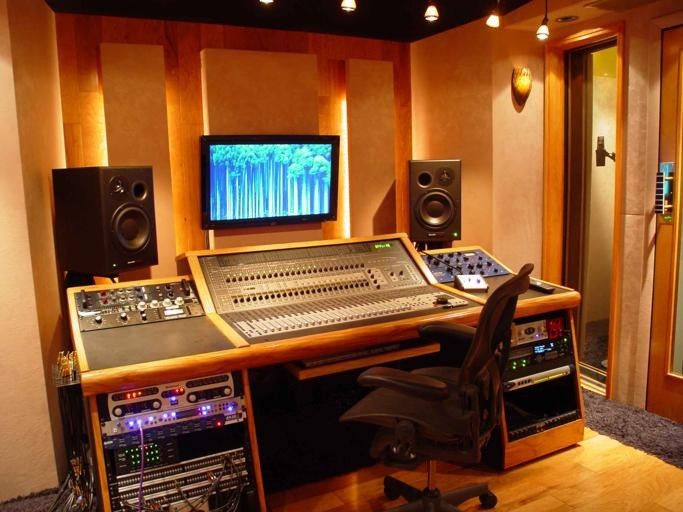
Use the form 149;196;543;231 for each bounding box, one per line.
200;134;341;229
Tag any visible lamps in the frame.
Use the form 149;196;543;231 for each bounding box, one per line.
512;63;532;107
259;0;551;41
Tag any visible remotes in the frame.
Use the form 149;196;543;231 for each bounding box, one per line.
529;279;556;293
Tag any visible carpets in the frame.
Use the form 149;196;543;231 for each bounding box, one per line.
582;388;683;471
0;487;62;512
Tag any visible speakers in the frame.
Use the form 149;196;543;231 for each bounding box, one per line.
408;158;462;242
52;166;159;276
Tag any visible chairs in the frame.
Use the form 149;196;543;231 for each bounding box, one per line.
339;262;535;512
